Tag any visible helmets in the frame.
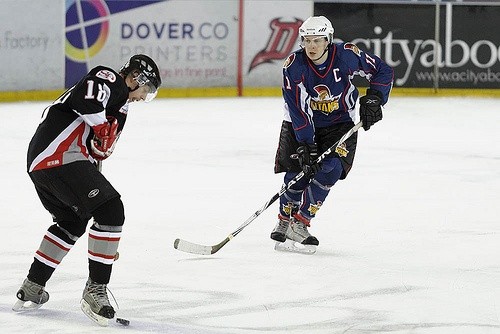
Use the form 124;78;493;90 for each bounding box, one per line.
118;54;162;93
298;16;334;42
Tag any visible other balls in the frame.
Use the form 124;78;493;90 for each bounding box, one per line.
116;317;131;326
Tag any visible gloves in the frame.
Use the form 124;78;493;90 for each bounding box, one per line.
290;142;324;181
88;115;122;161
359;89;383;131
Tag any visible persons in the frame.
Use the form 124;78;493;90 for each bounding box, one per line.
270;15;395;245
16;54;161;318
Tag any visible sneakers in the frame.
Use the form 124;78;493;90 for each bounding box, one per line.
9;278;50;313
273;218;319;256
79;277;117;327
270;217;290;243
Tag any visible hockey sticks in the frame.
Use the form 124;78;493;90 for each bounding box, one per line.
93;140;103;173
174;121;362;256
